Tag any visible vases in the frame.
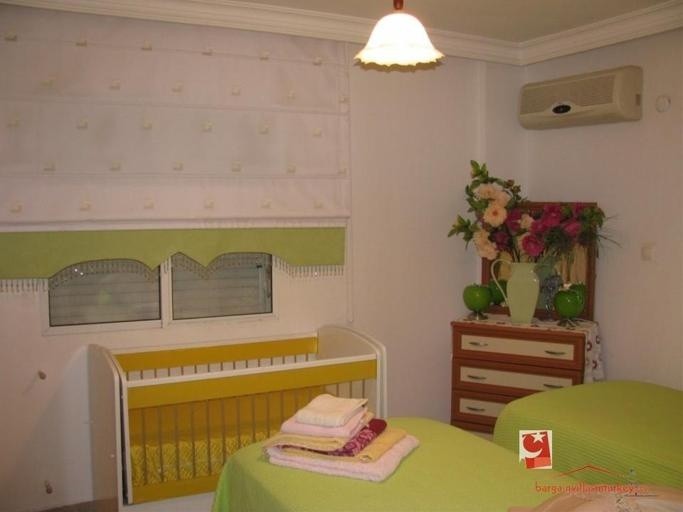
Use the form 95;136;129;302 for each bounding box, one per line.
491;259;540;325
533;257;557;309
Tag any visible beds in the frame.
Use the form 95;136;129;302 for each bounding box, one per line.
212;414;590;511
493;379;683;490
87;322;388;512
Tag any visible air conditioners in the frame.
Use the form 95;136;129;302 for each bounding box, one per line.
518;66;643;130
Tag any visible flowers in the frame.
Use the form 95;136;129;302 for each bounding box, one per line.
448;159;622;312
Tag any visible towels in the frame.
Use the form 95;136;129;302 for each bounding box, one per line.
260;391;420;483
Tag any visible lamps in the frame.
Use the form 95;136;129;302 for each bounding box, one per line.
352;1;446;68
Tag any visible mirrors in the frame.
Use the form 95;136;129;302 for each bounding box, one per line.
481;202;598;320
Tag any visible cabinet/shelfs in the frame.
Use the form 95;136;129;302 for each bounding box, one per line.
450;313;599;434
